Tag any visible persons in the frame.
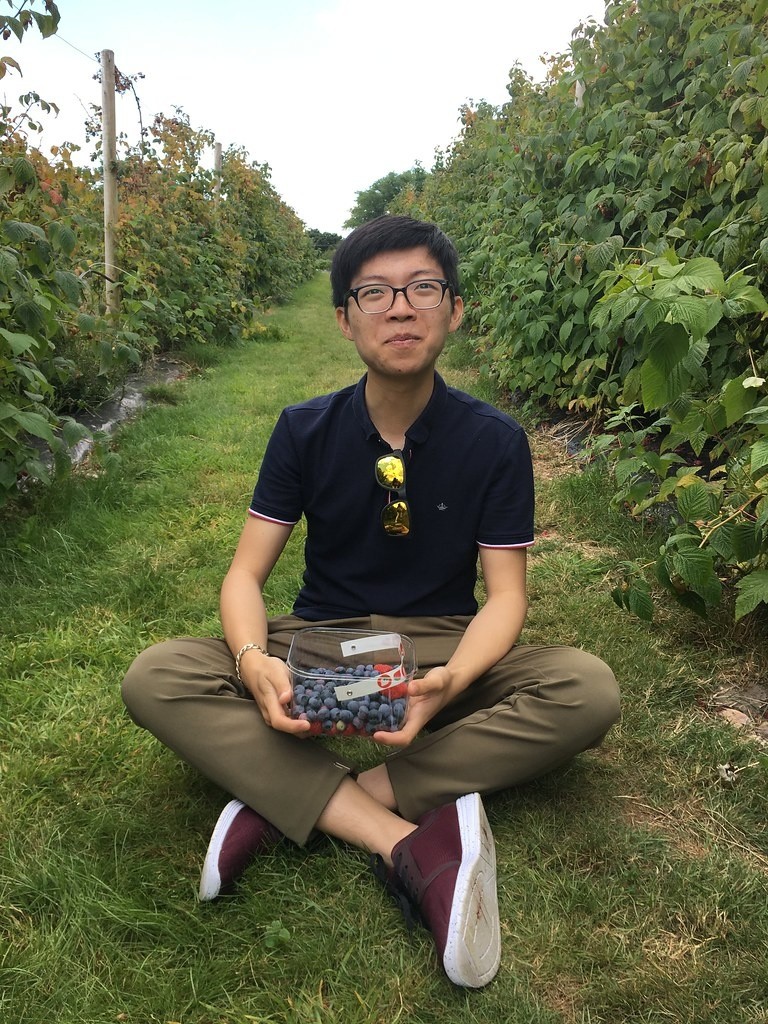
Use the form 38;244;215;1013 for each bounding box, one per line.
118;217;624;987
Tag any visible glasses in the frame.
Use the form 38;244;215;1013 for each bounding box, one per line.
373;448;412;540
344;277;451;317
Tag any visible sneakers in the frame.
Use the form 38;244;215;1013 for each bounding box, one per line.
368;790;501;990
198;801;285;901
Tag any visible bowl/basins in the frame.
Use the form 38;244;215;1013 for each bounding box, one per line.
285;626;419;737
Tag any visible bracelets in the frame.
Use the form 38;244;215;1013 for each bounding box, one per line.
235;644;270;687
